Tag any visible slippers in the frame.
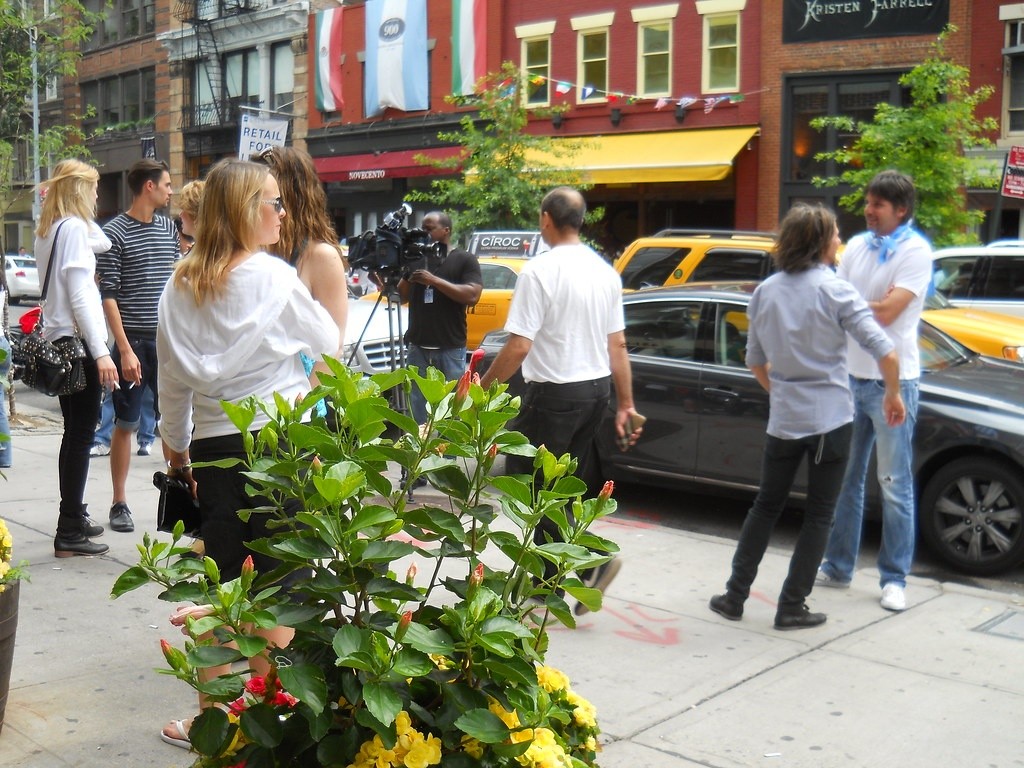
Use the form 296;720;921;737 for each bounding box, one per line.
160;718;201;753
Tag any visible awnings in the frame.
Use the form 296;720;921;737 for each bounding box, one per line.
466;129;760;185
302;147;463;182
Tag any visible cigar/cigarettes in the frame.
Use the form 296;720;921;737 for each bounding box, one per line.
113;380;121;390
129;381;136;389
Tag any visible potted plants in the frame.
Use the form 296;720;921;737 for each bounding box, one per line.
136;120;153;135
95;124;112;140
113;123;134;137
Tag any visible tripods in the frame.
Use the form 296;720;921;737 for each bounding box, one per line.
346;264;412;481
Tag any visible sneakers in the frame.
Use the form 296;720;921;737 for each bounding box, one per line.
89;441;111;457
574;550;621;617
136;442;152;454
527;576;564;604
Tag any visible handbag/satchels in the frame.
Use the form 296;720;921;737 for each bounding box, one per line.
10;325;87;398
152;470;203;540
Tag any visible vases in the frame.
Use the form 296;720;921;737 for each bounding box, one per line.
0;573;19;734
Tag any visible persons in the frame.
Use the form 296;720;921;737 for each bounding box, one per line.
474;186;645;616
93;145;350;755
18;247;31;258
810;169;934;611
709;202;905;630
0;281;13;468
377;210;482;490
37;159;121;557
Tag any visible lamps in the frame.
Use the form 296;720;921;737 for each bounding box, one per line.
420;110;446;147
610;108;623;125
674;105;688;121
324;121;352;154
551;113;565;128
364;118;395;156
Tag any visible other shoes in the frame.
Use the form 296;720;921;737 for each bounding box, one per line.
399;475;424;490
880;582;905;611
179;538;206;558
813;567;852;588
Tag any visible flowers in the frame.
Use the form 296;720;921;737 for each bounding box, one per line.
0;519;31;592
110;352;617;768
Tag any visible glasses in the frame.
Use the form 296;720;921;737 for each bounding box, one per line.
249;196;283;214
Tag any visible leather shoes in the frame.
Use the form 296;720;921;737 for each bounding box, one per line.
109;502;134;532
79;514;104;537
774;604;827;630
53;531;109;558
710;594;744;621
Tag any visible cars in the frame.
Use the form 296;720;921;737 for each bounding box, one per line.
469;280;1024;575
336;296;408;381
0;252;48;306
354;253;537;351
604;226;1023;377
915;238;1024;323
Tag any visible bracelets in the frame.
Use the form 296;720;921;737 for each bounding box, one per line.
168;458;192;473
